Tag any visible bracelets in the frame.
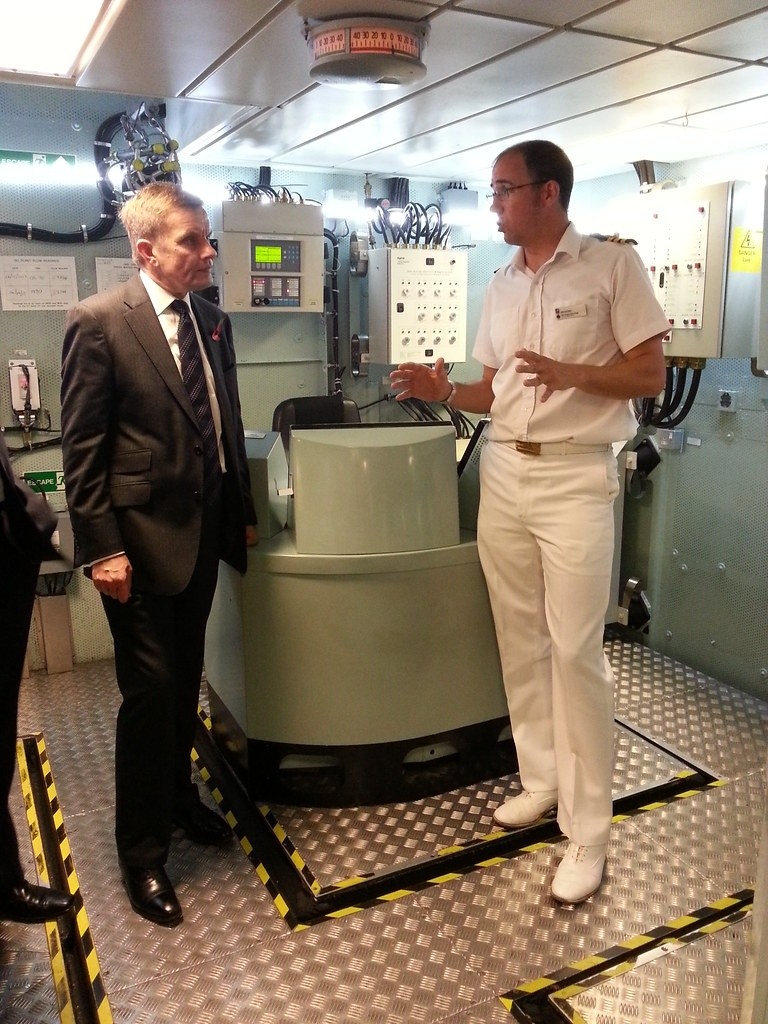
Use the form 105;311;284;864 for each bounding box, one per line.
440;380;457;405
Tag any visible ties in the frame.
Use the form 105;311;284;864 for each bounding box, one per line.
170;299;222;507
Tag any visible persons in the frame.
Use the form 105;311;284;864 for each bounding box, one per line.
0;426;78;926
61;183;260;927
391;142;672;908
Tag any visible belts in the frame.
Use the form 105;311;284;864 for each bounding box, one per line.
495;437;611;455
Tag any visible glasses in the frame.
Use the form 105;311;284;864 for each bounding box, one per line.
486;178;550;204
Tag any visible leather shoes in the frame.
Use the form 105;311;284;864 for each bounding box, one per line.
177;800;237;848
493;786;558;826
551;839;610;903
118;852;182;922
0;879;73;924
